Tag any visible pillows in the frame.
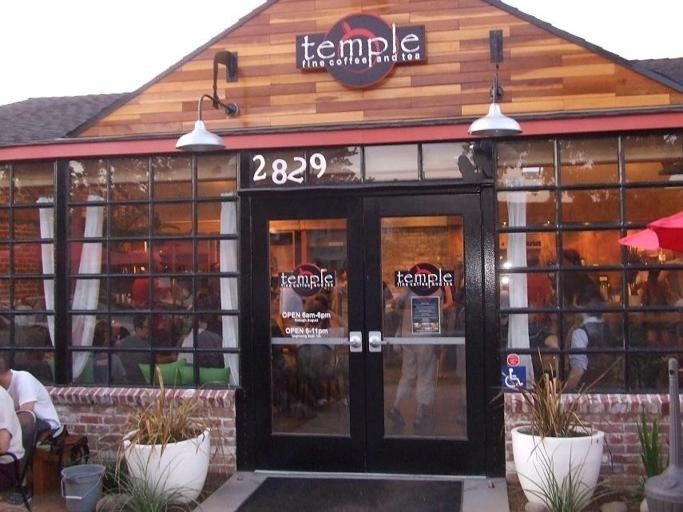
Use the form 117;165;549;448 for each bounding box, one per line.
138;361;230;388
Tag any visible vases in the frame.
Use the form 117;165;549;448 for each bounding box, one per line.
122;427;211;505
511;426;604;512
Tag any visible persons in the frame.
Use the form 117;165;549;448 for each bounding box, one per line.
92;262;224;385
500;249;683;389
387;263;467;429
271;268;348;407
0;351;63;507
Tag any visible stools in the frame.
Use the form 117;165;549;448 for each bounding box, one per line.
61;435;90;467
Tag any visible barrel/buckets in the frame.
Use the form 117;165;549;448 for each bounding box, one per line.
60;464;106;512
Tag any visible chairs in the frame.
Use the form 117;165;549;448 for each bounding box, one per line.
0;408;38;512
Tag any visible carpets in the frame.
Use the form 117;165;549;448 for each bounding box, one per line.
234;477;464;512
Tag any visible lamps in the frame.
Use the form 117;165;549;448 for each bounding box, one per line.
176;94;239;154
467;77;524;139
